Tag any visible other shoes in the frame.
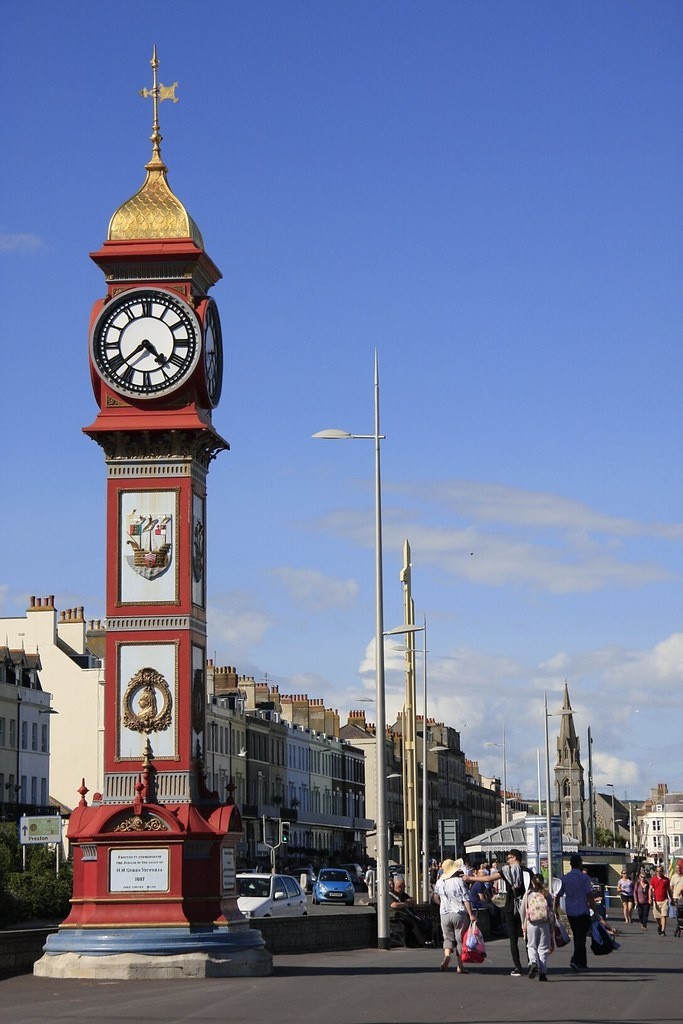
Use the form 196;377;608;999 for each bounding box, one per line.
641;924;644;930
539;972;548;982
580;966;589;971
569;960;580;973
440;956;451;971
511;969;521;977
529;964;538;979
661;931;665;937
657;926;661;936
644;926;648;931
457;968;470;974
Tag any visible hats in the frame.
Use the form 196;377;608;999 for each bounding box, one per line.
440;858;463;880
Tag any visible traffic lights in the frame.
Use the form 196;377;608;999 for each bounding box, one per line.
282;822;290;843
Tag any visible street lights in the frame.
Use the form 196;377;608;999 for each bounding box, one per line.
389;613;450;902
311;346;424;948
486;728;508;824
605;783;622;848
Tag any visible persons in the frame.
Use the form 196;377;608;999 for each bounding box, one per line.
365;866;375;899
308;858;328;874
387;848;683;983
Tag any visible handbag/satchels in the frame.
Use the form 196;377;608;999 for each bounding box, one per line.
514;896;524;915
555;919;571;948
461;919;487;963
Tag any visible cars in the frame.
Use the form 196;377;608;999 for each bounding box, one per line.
235;873;309;918
291;862;365;905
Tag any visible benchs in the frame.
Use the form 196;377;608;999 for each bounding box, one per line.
369;903;441;948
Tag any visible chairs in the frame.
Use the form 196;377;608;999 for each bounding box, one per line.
324;874;331;881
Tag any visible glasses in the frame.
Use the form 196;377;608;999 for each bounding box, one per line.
506;856;514;861
656;871;662;872
622;873;626;874
641;876;646;879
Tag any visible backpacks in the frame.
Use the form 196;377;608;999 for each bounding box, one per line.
527;891;549;922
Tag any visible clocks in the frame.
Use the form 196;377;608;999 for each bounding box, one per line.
89;286;202;399
194;296;223;408
